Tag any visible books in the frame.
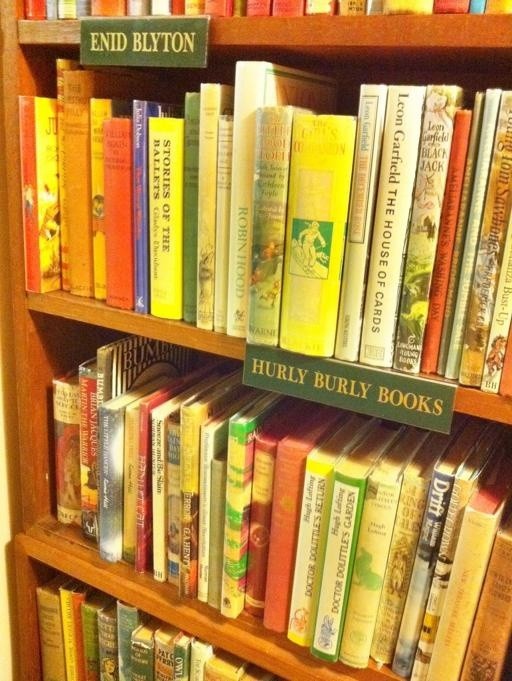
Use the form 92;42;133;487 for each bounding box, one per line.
51;335;512;678
37;572;280;681
18;0;512;396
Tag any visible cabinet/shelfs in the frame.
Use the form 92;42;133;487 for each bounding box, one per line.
0;0;511;680
